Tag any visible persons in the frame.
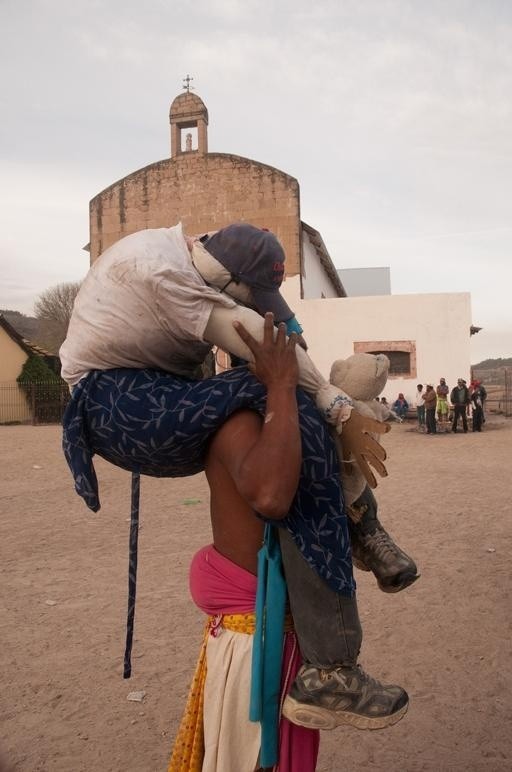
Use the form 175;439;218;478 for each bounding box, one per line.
60;225;421;730
166;311;319;771
375;376;487;433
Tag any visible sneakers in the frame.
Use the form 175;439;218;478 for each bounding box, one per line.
280;653;412;735
348;517;423;596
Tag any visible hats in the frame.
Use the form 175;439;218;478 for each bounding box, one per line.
202;220;296;326
